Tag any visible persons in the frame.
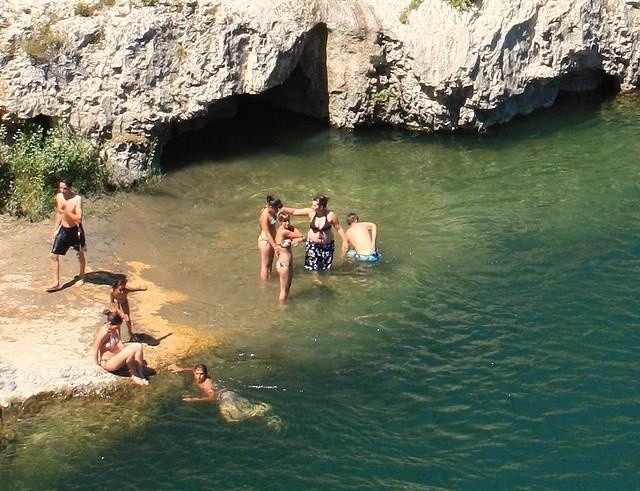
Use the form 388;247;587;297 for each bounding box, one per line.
342;212;381;263
93;310;149;385
273;211;308;300
110;277;149;342
166;362;269;424
277;194;349;273
46;177;87;293
256;193;282;281
